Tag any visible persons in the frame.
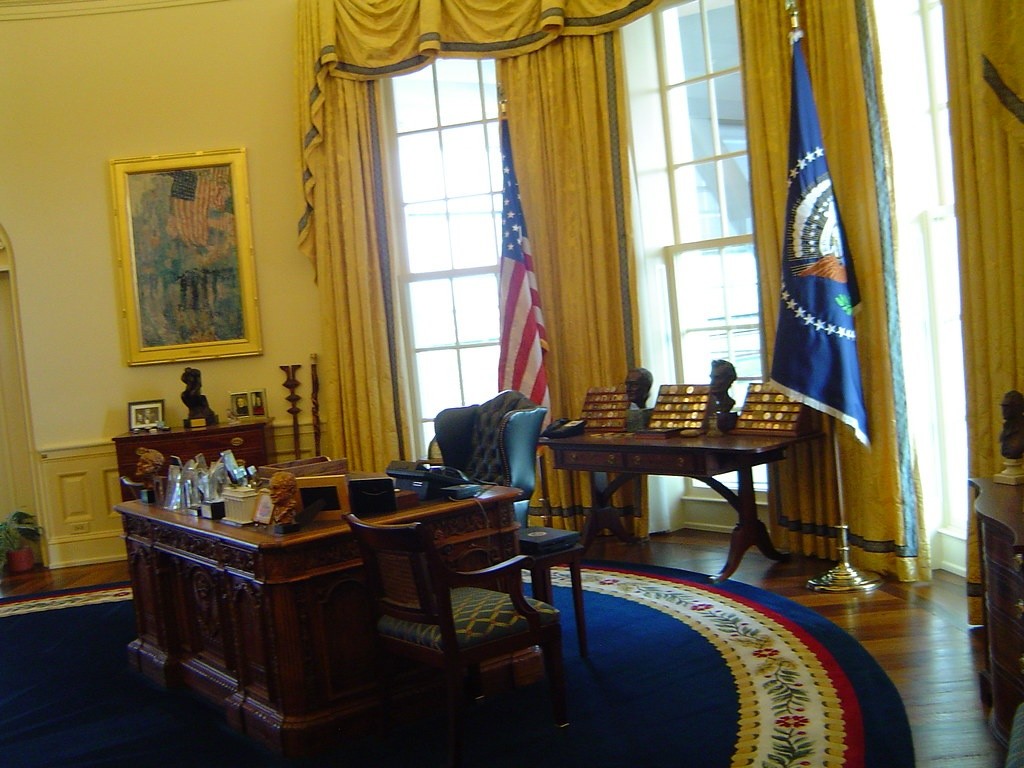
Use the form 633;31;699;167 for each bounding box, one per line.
236;397;247;414
253;396;264;414
135;448;164;492
268;472;297;526
710;360;736;414
627;367;653;410
1000;391;1024;459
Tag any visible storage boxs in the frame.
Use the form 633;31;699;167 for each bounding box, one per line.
516;526;580;555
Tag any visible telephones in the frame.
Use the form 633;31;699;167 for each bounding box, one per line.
542;414;586;442
387;457;468;500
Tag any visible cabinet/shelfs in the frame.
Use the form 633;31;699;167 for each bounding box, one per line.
968;476;1024;750
112;417;282;501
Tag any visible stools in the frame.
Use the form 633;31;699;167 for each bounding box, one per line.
522;543;588;659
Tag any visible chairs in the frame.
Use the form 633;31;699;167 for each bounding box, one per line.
433;386;550;528
342;514;571;768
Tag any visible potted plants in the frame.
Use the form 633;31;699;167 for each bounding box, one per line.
0;512;44;579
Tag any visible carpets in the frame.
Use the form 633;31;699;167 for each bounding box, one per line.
0;558;920;768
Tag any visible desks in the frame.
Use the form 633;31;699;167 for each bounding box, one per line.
540;424;826;581
112;471;547;761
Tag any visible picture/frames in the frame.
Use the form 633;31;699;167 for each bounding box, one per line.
127;399;166;432
110;146;265;367
230;389;268;418
294;473;349;522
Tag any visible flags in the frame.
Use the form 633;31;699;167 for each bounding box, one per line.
499;118;551;481
768;29;879;448
